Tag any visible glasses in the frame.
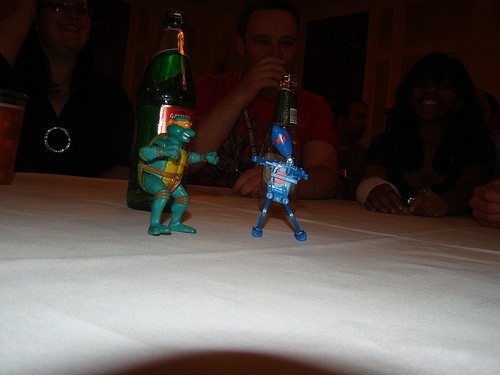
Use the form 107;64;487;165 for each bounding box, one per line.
38;0;94;17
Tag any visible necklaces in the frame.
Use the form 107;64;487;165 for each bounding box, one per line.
48;61;73;99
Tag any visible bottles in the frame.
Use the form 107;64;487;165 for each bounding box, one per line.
126;10;196;211
259;74;301;216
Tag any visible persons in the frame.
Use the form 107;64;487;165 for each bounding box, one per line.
356;52;495;218
469;177;500;229
0;0;135;180
328;92;500;201
189;0;339;200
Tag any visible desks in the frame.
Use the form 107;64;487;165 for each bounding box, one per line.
0;174;500;375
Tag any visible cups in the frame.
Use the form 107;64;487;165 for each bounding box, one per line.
0;103;25;185
